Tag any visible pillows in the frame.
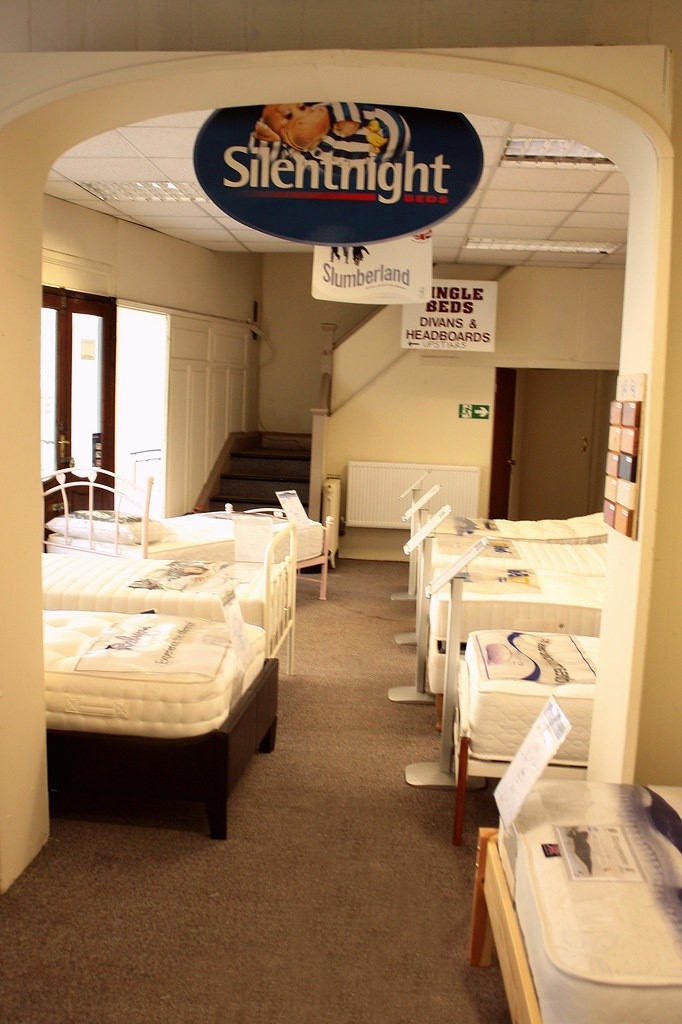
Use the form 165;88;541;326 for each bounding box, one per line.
46;510;171;546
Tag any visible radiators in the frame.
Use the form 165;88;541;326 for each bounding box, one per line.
322;473;342;568
342;461;481;529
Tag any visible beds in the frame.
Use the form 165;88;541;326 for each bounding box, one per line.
389;488;607;846
40;467;334;600
470;782;681;1024
44;524;297;677
43;609;279;842
